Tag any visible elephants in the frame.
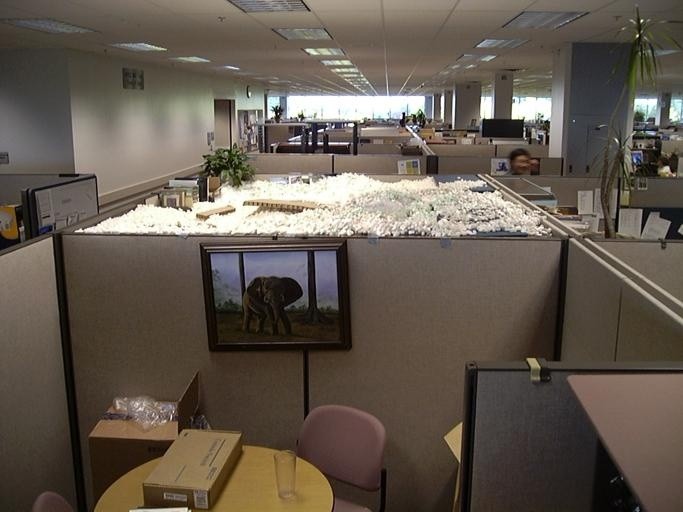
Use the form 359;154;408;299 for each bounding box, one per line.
241;275;303;338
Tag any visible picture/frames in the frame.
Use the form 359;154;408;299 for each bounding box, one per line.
197;236;352;353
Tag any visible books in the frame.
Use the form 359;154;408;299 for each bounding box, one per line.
550;210;605;239
159;178;201;212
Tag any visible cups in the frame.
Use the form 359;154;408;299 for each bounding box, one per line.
273;449;297;499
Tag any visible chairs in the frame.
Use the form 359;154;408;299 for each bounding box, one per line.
295;404;388;512
32;490;76;512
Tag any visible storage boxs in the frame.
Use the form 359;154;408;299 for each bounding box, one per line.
86;371;199;506
444;420;464;510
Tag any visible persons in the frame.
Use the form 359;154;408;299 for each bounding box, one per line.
649;157;674;177
506;146;540;176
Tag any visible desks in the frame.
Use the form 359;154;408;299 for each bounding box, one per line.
94;445;334;512
